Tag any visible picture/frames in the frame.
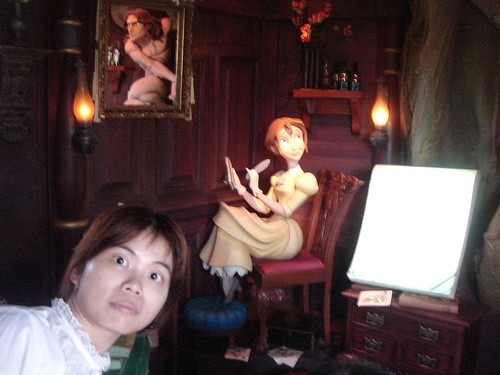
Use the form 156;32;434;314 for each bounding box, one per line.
94;0;194;119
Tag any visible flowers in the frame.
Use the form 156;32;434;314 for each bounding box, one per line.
288;0;352;43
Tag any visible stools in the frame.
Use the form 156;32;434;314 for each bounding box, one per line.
183;296;248;349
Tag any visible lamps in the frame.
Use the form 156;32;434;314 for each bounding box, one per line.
371;75;390;128
72;56;99;154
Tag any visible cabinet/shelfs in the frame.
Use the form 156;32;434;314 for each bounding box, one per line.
338;288;491;375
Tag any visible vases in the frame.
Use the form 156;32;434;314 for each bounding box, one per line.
302;42;319;88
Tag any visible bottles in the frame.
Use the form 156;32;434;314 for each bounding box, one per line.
339;65;349;89
349;62;360;90
331;71;339;88
320;54;333;88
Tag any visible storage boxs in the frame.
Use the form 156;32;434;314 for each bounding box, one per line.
269;312;322;352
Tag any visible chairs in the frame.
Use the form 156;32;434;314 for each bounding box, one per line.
254;170;363;354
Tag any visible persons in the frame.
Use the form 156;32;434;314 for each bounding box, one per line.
200;117;319;305
123;8;177;106
0;204;187;375
